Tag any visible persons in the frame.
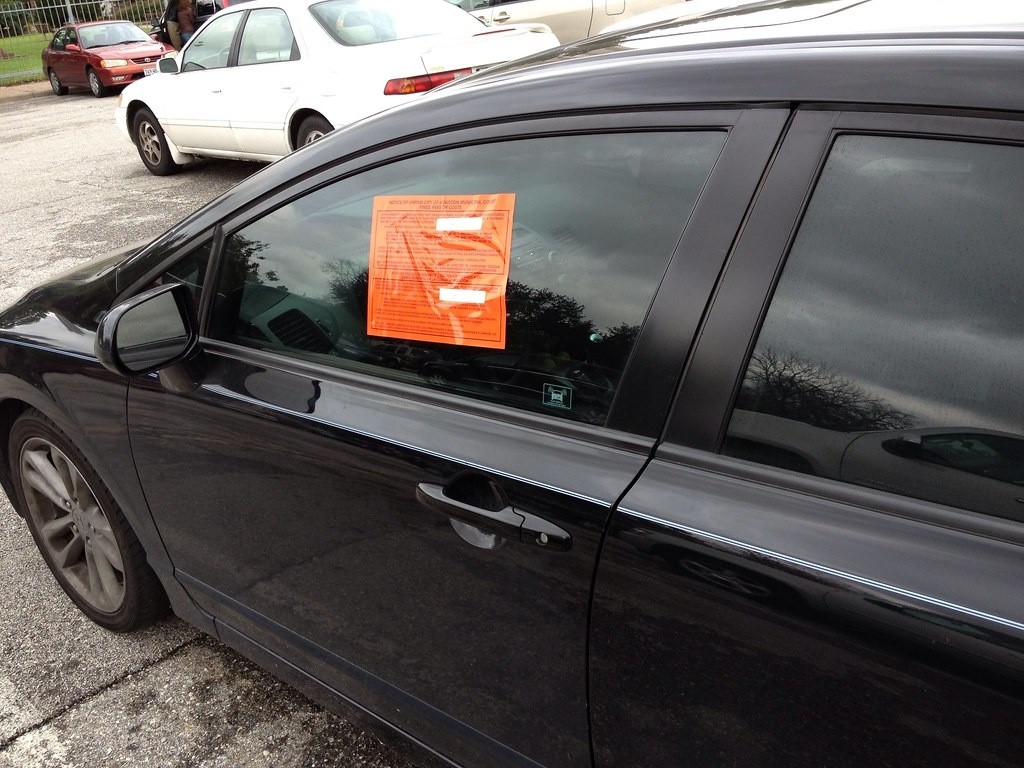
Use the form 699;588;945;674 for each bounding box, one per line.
166;0;195;52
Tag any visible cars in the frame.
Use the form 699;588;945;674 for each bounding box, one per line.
0;1;1024;768
113;0;562;178
445;0;682;45
40;19;179;97
150;0;259;50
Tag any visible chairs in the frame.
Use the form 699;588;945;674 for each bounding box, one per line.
93;33;106;43
75;36;89;48
237;42;257;61
